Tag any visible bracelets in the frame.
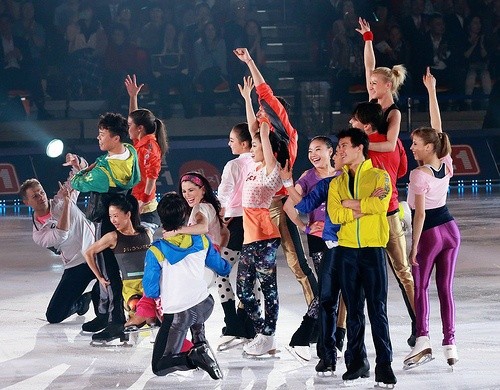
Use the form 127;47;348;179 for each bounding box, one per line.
303;225;311;234
77;155;81;164
259;117;269;124
282;178;293;187
246;58;253;64
141;193;152;202
97;275;103;280
363;31;373;41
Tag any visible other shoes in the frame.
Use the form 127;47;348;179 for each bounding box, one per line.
76;292;92;316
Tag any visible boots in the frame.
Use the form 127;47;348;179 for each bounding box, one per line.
285;314;317;367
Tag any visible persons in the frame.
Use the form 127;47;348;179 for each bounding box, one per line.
282;136;344;361
141;192;232;380
0;0;499;133
328;129;398;384
333;101;431;352
19;153;100;323
354;15;414;237
124;74;172;244
83;194;158;331
134;173;230;352
277;141;370;378
236;76;287;356
403;66;461;365
215;121;263;336
69;114;142;343
225;48;320;339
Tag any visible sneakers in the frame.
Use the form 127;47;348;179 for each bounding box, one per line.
89;323;134;348
407;331;431;350
398;200;413;234
342;357;371;385
315;351;338;378
442;344;459;372
373;362;397;389
217;325;252;352
124;298;157;333
242;332;281;361
186;342;224;381
333;328;345;359
80;313;111;338
402;335;435;371
